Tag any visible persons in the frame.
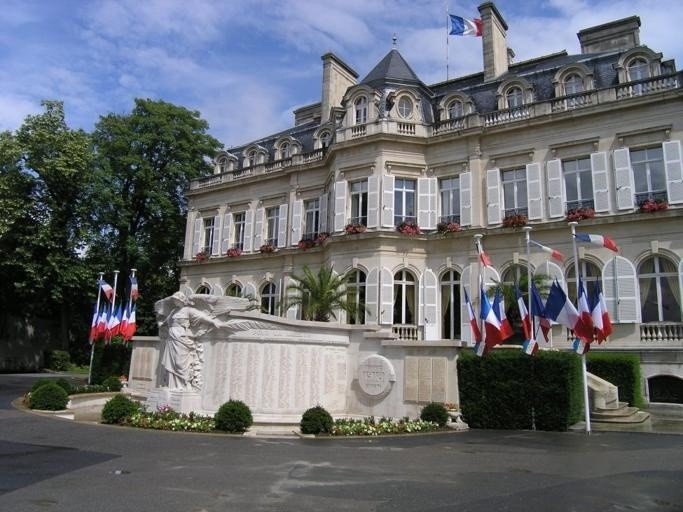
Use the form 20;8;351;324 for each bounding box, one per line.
158;291;222;394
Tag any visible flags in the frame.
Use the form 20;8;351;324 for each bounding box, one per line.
449;14;481;36
463;233;618;359
88;277;138;346
